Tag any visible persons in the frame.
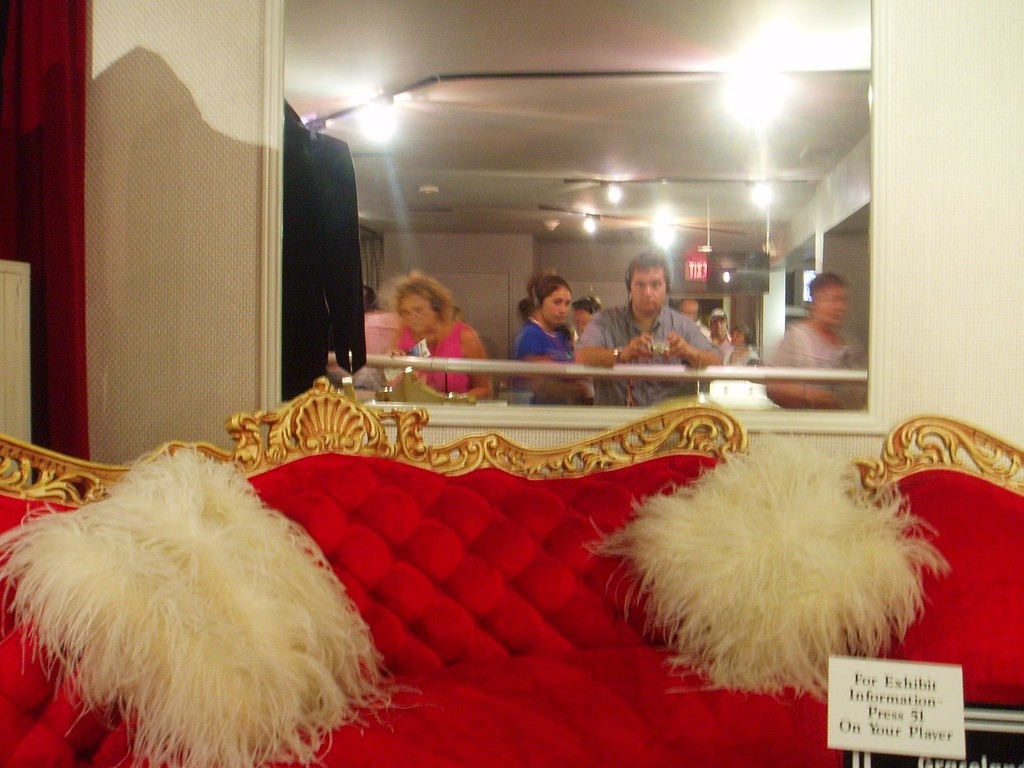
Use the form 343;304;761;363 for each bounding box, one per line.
379;270;492;404
282;99;376;401
512;252;760;407
765;272;865;410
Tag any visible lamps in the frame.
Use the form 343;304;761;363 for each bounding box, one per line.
582;213;602;233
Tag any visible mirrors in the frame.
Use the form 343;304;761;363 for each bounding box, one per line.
254;0;891;439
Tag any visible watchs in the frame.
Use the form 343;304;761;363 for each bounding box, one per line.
614;344;627;364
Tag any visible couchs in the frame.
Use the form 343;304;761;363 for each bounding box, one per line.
0;375;1024;768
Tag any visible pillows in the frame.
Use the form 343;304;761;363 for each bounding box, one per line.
0;441;437;768
576;436;949;701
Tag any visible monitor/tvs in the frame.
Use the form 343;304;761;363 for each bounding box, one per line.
706;252;770;292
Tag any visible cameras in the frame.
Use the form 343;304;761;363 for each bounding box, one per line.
648;339;670;355
